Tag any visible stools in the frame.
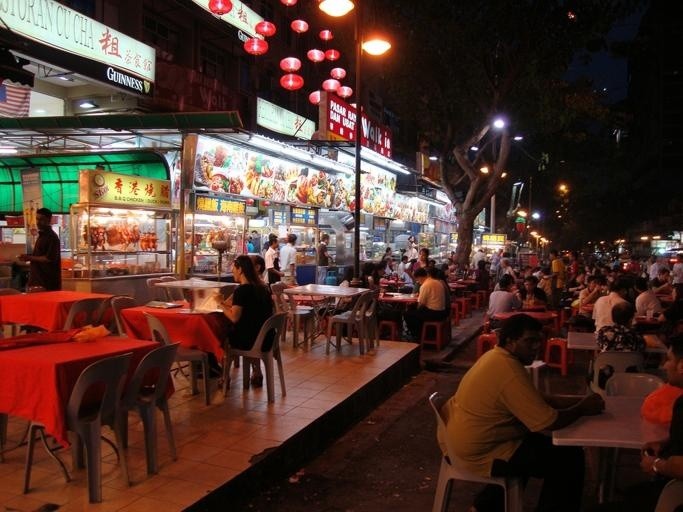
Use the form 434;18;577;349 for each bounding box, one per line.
527;361;552;396
543;338;575;376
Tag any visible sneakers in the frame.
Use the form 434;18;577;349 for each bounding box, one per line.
250;375;264;388
216;379;232;391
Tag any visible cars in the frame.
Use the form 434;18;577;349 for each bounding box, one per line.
557;247;682;269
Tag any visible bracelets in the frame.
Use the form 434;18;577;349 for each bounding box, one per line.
653;458;660;472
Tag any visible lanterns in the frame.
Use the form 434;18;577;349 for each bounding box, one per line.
208;0;232;16
244;38;270;56
255;21;278;37
280;0;353;107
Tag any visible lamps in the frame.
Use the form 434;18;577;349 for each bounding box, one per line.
78;100;100;111
59;74;74;82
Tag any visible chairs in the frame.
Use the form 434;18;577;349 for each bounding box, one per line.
222;311;287;405
430;392;526;511
606;372;664;502
23;352;136;505
589;351;645;402
2;252;682;356
129;341;184;478
144;312;218;405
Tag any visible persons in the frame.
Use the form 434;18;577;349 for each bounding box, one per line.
18;208;62;291
317;235;333;285
442;313;605;512
471;249;683;374
639;334;683;512
335;246;451;335
218;255;275;386
248;230;299;286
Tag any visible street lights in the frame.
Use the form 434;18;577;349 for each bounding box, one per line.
515;208;552;257
479;166;506;237
490;113;525;162
318;1;392;333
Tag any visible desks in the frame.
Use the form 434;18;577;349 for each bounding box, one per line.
550;395;646;449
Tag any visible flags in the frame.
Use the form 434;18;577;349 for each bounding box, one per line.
0;81;32;117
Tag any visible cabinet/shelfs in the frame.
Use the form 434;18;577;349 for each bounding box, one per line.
66;200;459;280
